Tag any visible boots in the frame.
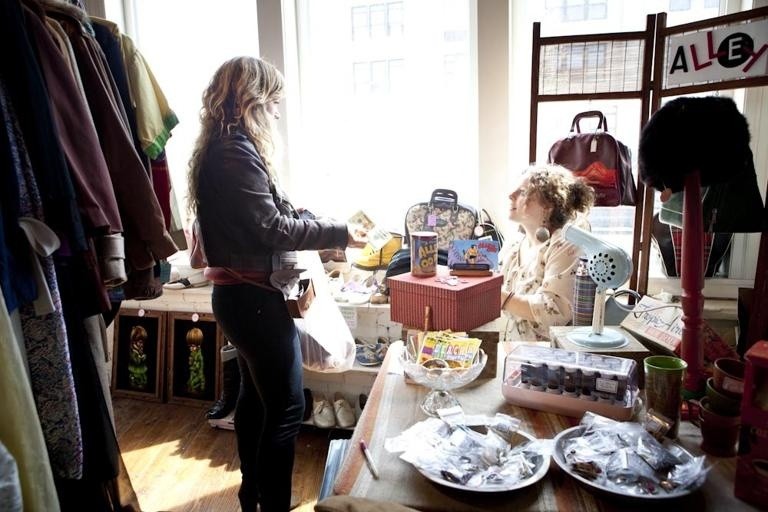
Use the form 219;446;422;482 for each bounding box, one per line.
205;345;241;419
351;233;402;270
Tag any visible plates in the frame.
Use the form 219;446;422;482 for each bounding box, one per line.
412;416;553;496
551;421;709;500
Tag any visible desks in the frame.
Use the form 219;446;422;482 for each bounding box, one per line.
333;339;768;512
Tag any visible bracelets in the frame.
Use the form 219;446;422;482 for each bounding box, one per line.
501;292;515;310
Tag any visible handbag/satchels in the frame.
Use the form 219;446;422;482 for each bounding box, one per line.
190;220;209;267
549;110;637;206
404;189;504;277
269;267;316;319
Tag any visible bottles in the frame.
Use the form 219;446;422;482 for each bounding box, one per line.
410;231;438;279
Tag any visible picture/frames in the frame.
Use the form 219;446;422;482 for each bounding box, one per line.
167;311;224;408
110;307;166;404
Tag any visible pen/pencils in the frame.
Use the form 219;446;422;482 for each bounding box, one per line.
360;439;379;478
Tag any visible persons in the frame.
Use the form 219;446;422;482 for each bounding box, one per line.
186;327;205;397
490;163;595;341
128;325;148;391
185;57;370;512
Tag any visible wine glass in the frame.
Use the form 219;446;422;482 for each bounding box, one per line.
396;340;488;416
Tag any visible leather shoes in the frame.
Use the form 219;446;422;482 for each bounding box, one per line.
325;269;391;305
301;388;368;427
163;270;208;290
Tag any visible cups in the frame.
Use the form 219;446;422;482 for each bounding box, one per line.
697;355;745;458
643;355;689;441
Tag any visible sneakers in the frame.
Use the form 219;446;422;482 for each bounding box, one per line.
355;335;390;366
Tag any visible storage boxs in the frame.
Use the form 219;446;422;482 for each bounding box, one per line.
386;265;504;331
